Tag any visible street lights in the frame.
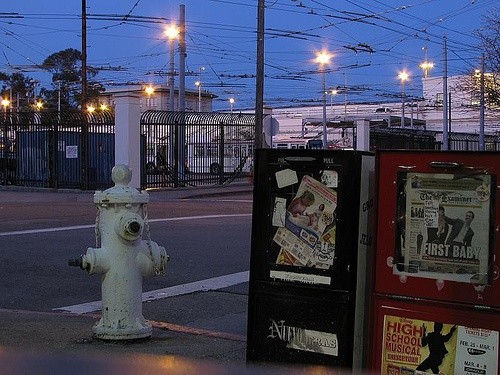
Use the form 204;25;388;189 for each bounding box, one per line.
421;62;432;78
400;72;407;128
145;88;154;109
195;82;203;112
2;100;10;185
230;97;234;110
165;23;179;166
318;53;330;150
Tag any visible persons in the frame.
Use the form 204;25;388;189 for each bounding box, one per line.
312;204;324;226
416;230;424;255
415;322;457;375
442;211;475;259
289;191;314;218
411;205;424;218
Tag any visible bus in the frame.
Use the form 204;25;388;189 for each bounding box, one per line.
146;137;169;174
185;137;323;174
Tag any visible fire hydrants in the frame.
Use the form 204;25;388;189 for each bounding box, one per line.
68;165;170;340
